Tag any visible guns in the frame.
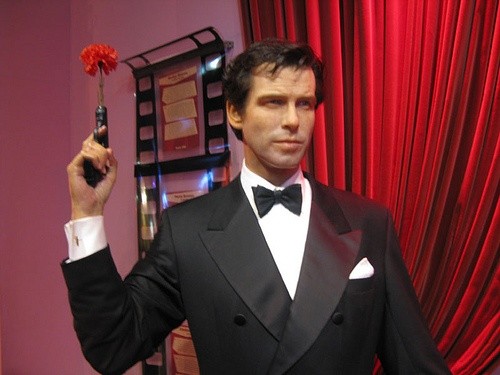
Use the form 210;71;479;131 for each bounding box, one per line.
82;105;109;187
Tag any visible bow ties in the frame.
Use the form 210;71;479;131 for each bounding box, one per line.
252;184;303;218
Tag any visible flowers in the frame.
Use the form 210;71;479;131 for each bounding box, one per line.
79;44;119;105
